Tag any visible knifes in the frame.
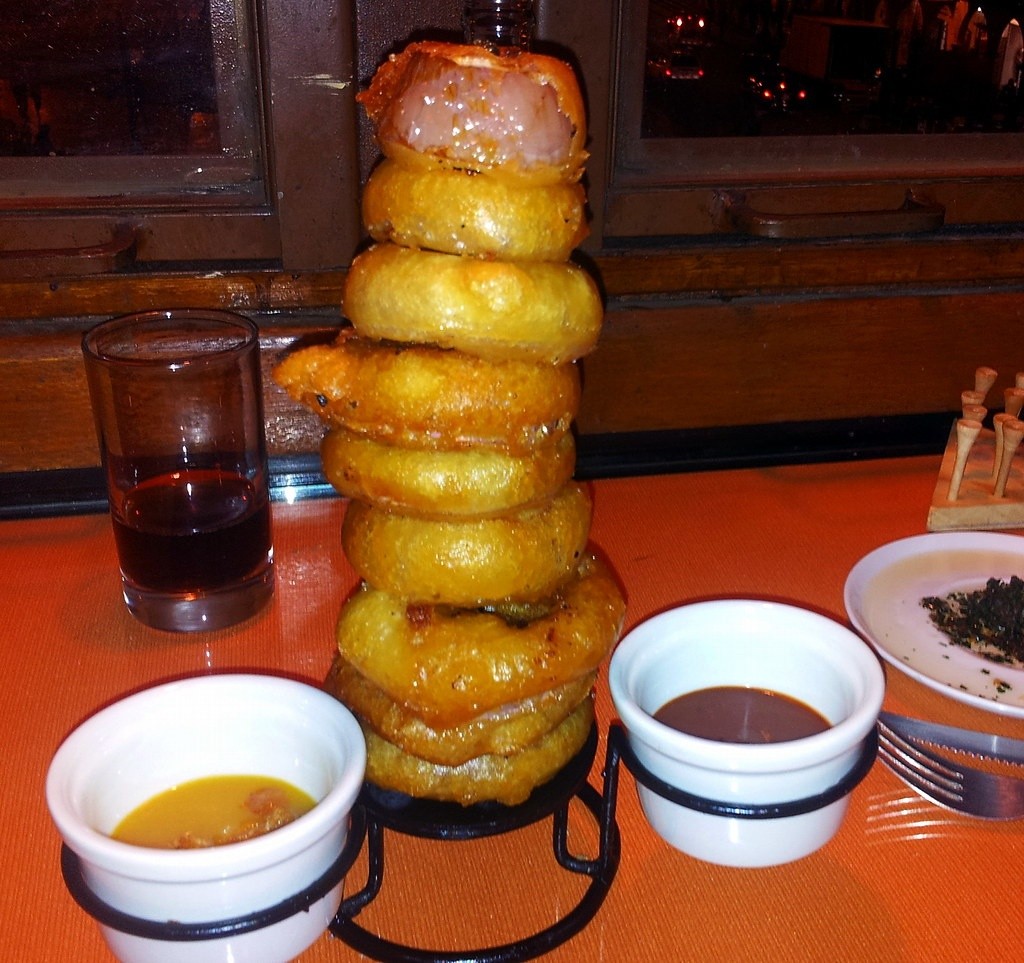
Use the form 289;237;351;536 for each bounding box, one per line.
879;712;1024;768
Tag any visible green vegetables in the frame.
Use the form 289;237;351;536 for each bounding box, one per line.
916;570;1024;696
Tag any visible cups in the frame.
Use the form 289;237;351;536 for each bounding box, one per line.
82;308;274;634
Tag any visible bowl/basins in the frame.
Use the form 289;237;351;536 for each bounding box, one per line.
44;674;366;963
607;599;886;868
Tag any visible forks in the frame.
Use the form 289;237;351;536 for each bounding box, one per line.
868;788;972;847
877;717;1024;824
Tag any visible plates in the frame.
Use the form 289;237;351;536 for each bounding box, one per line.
843;531;1024;720
